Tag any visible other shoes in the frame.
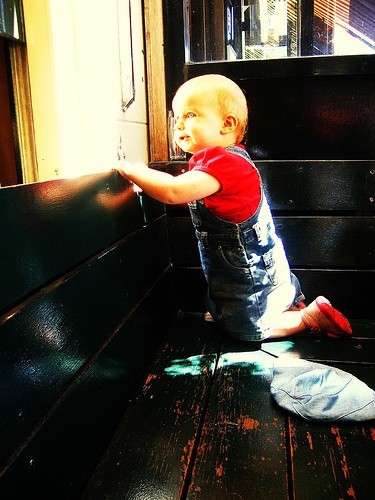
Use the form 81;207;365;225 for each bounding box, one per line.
301;296;353;339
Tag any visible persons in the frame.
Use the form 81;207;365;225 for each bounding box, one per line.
109;72;353;344
